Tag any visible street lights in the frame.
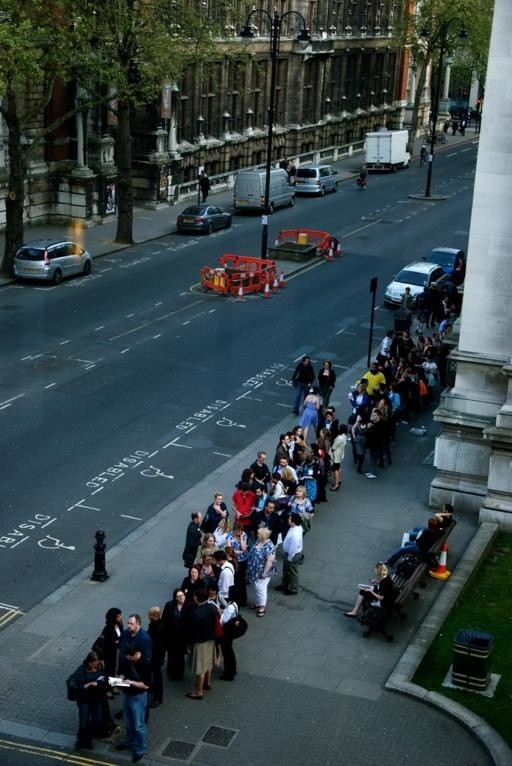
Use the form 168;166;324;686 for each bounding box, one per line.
422;15;469;195
241;10;314;271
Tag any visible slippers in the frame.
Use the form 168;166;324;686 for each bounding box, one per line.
249;603;260;609
257;610;265;617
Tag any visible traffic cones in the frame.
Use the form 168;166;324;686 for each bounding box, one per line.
335;242;343;256
270;275;280;291
326;248;334;261
262;278;272;297
234;280;247;302
331;240;336;253
278;269;286;288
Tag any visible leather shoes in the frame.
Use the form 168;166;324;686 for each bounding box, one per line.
133;753;143;762
185;692;202;699
194;682;211;690
107;694;114;699
150;701;158;708
274;584;288;590
283;589;297;595
113;691;120;695
343;613;357;618
115;743;127;750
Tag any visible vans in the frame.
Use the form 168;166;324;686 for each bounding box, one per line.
234;167;295;212
295;165;341;196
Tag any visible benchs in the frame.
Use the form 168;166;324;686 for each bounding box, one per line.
391;520;457;586
357;559;428;643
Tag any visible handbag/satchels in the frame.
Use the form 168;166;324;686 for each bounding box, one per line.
67;673;83;700
223;615;248;639
394;552;419;579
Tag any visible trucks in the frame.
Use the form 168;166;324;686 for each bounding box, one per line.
362;129;412;173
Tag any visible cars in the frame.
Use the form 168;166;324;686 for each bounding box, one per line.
424;247;466;287
176;202;233;233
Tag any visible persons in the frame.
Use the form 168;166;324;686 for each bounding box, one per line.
200;173;210;202
280;156;288;169
75;280;459;764
360;165;368;190
443;119;468;136
287;159;296;184
420;145;427;168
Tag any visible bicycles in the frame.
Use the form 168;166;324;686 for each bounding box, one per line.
424;129;448;145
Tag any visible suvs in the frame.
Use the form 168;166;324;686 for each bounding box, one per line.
9;237;91;284
384;260;446;309
